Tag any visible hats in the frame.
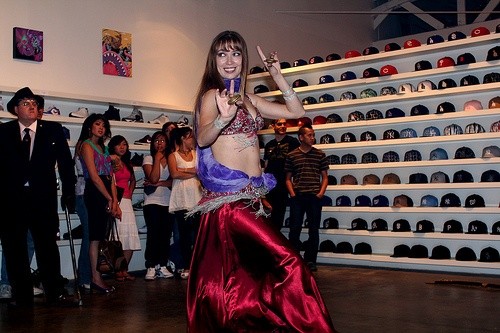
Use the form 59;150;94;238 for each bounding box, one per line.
250;23;500;264
6;87;45;116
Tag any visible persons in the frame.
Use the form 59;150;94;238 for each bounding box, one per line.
187;31;336;333
0;87;79;308
107;135;142;279
74;113;122;293
262;118;301;232
285;126;329;273
142;131;174;278
162;122;204;277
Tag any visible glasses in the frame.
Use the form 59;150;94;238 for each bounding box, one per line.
18;101;39;108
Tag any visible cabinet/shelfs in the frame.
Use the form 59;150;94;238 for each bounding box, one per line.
246;18;500;278
0;84;197;280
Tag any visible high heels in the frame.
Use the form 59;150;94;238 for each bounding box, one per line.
90;281;115;294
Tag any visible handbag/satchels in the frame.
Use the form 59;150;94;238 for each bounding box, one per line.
96;218;127;273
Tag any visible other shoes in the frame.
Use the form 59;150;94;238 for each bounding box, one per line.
308;261;317;272
63;226;83;239
180;272;189;278
115;275;135;281
53;295;79;307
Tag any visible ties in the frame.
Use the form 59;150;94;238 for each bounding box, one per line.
23;128;30;162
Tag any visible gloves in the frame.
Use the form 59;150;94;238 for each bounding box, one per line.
61;190;76;213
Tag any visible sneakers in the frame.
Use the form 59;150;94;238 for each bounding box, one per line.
134;135;152;144
145;268;157;280
138;225;147;233
122;108;144;123
157;266;174;279
148;113;168;125
103;105;121;120
44;107;60;115
70;107;88;117
131;152;143;167
177;116;189;126
132;200;144;211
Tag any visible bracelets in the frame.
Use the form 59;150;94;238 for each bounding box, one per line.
282;86;296;100
213;114;228;129
